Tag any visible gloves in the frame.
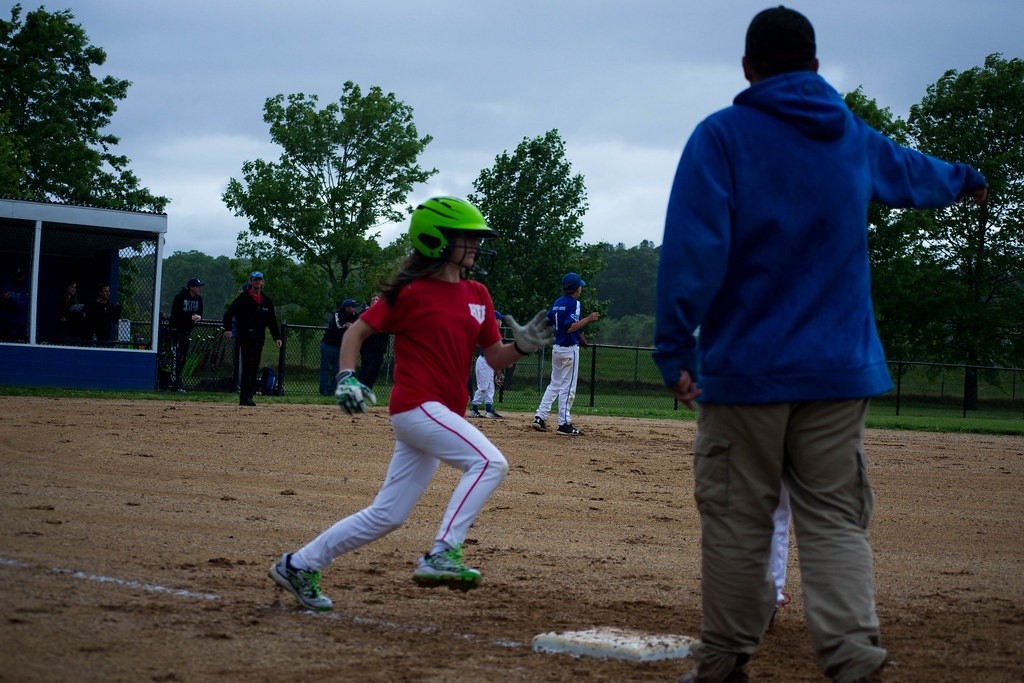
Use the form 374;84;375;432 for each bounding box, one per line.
336;369;378;416
506;310;556;355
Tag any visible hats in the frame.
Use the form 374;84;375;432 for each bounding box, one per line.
344;299;359;307
745;5;816;65
187;279;205;288
494;311;506;319
562;273;585;289
250;271;263;280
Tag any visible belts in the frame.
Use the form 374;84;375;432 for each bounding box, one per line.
553;343;569;347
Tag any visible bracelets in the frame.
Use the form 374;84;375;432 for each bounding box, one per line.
514;341;530;355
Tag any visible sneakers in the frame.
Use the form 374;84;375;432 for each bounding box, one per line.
532;416;546;431
468;410;484;418
413;543;480;588
268;552;333;611
485;410;503;419
556;424;582;435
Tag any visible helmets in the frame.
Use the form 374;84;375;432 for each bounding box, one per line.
409;196;498;259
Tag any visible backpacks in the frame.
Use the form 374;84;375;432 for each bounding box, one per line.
258;366;277;395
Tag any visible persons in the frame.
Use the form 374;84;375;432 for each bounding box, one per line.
85;283;122;346
58;281;86;336
0;267;31;338
223;271;282;405
767;481;792;630
355;295;389;390
320;298;359;397
267;198;556;611
471;309;506;418
231;282;251;392
650;6;988;682
533;272;600;435
170;278;205;392
